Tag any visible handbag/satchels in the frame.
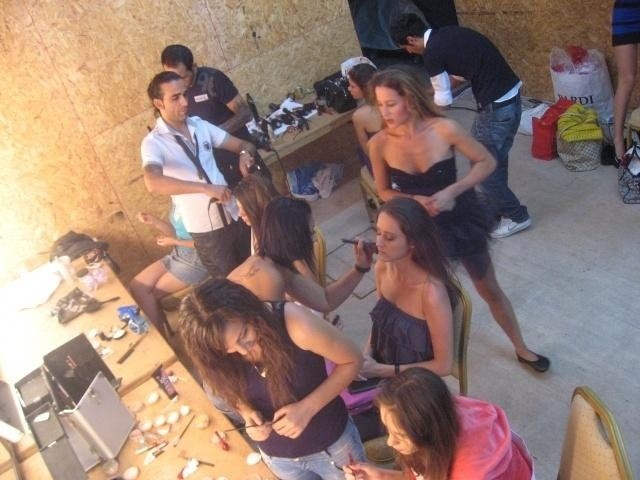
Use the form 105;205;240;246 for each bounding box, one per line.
617;142;640;204
348;353;386;395
532;96;604;171
550;46;614;120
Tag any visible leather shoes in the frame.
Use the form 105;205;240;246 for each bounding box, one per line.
516;350;549;372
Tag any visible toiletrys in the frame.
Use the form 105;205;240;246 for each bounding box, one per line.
53;255;72;281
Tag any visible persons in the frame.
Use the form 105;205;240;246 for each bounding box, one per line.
389;13;532;238
131;44;274;339
177;171;533;479
346;64;391;209
612;0;640;169
367;67;550;372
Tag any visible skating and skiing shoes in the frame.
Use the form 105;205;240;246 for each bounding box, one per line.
489;216;532;239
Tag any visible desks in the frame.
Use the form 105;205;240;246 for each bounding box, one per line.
254;95;365;198
0;249;178;473
0;360;280;480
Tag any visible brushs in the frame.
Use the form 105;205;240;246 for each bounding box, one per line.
64;355;91;383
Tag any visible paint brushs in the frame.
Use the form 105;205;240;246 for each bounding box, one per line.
222;420;280;434
174;415;194;447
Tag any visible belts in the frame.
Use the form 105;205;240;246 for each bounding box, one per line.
487;92;520;109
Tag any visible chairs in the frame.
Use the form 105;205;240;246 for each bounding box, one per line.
627;106;640;150
362;273;473;465
558;384;632;480
311;225;328;326
158;275;196;337
357;164;386;223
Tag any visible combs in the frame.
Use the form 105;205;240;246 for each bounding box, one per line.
117;333;147;364
85;296;120;313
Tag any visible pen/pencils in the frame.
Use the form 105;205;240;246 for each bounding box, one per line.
349;452;356;477
